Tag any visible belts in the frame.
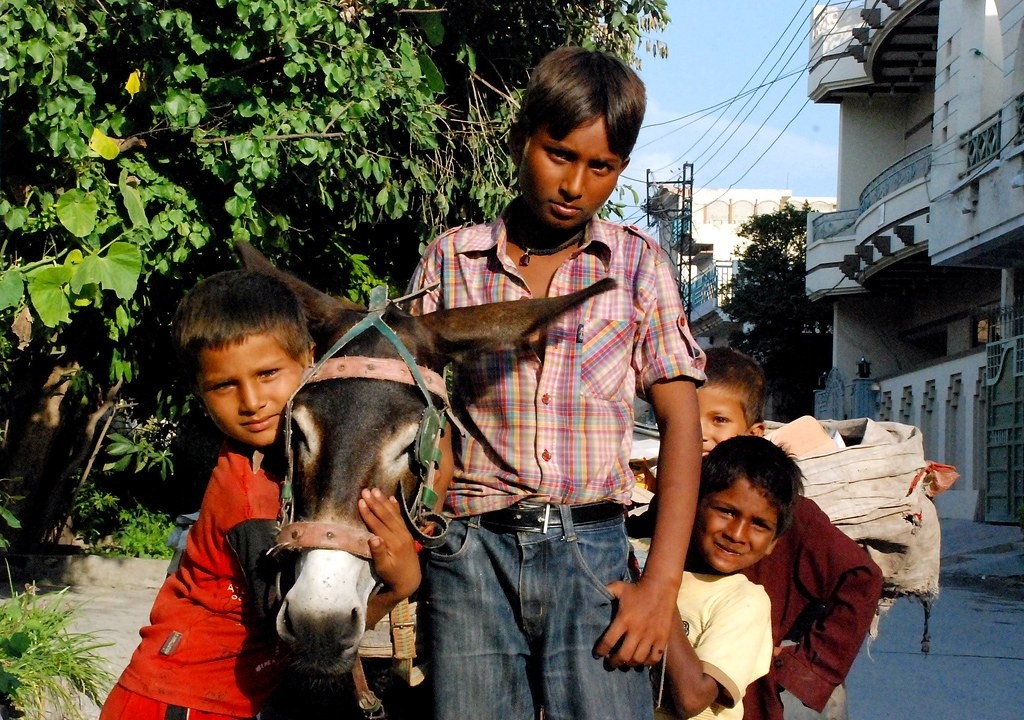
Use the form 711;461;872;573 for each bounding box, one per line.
464;501;623;531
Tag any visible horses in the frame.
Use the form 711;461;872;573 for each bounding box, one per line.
232;237;619;720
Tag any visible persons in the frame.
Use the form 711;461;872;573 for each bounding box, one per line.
100;267;423;720
635;434;810;720
627;342;882;718
396;44;710;720
